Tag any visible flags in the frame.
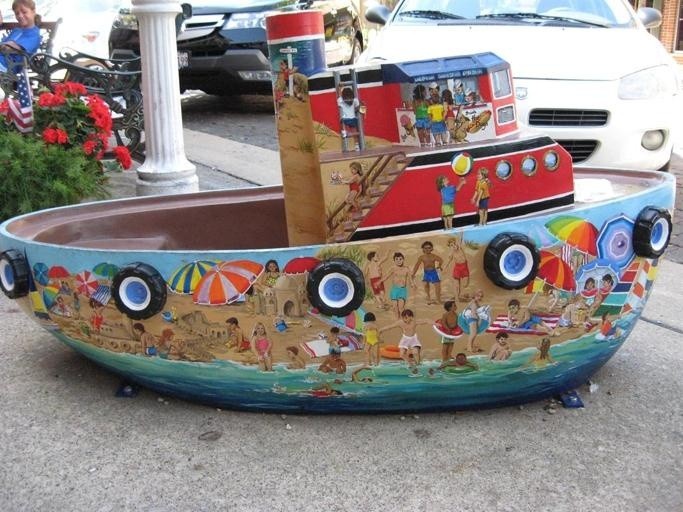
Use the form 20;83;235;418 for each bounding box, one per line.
0;66;34;135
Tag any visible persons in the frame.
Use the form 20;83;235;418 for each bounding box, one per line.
410;78;482;147
279;59;306;104
0;1;42;77
433;173;468;233
467;167;493;227
334;80;361;151
335;159;367;213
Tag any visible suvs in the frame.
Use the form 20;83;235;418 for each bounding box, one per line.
109;0;364;101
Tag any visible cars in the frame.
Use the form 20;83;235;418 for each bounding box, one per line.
353;1;681;185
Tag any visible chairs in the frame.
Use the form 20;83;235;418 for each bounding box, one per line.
0;16;150;174
